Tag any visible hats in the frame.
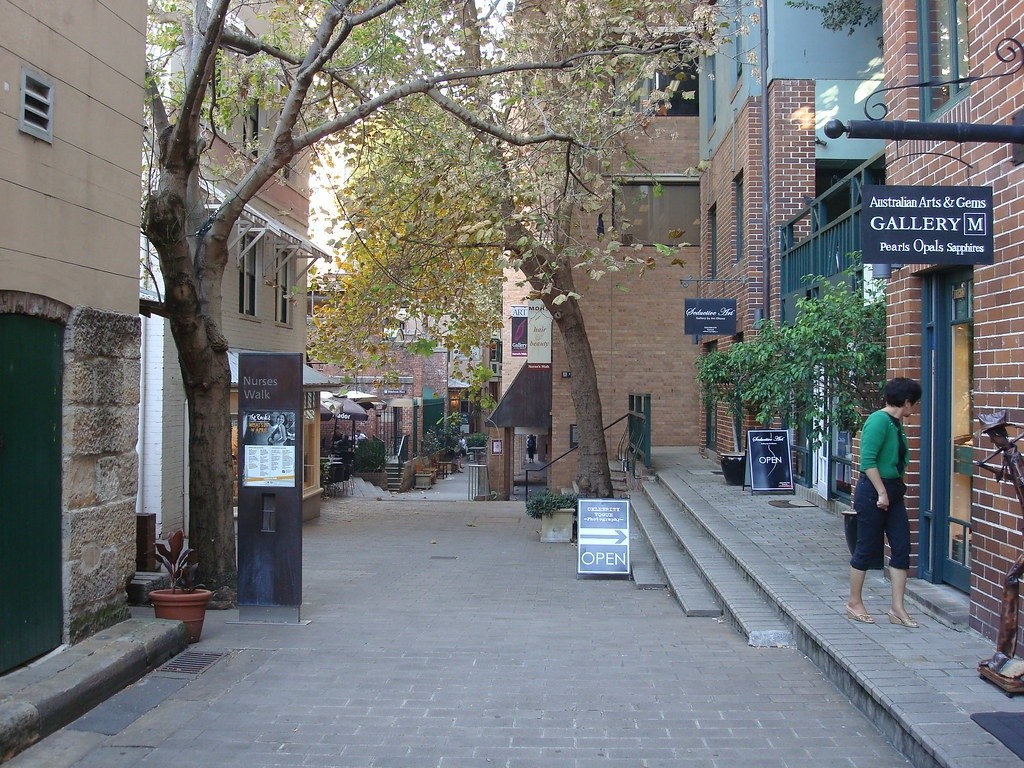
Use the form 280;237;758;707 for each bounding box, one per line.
357;430;362;433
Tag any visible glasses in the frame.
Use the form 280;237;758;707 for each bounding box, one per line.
914;399;921;405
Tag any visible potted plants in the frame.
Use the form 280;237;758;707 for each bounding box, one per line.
789;249;886;555
524;488;578;543
692;348;759;486
147;528;213;644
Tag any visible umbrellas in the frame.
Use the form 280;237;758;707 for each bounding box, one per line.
320;403;334;422
330;398;369;456
354;400;387;450
320;391;337;400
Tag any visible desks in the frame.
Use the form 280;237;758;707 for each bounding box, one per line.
320;457;343;461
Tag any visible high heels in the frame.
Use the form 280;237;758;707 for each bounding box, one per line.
888;612;919;628
846;607;874;624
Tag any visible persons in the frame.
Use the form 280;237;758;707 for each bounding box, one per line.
357;429;369;439
528;434;536;463
527;436;530;454
267;414;288;446
846;376;923;629
444;445;465;474
459;436;466;455
459;439;465;456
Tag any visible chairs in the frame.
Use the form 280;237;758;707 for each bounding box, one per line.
430;453;448;479
320;461;344;498
339;450;356;495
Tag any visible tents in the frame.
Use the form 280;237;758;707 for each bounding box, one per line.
343;391;379;402
